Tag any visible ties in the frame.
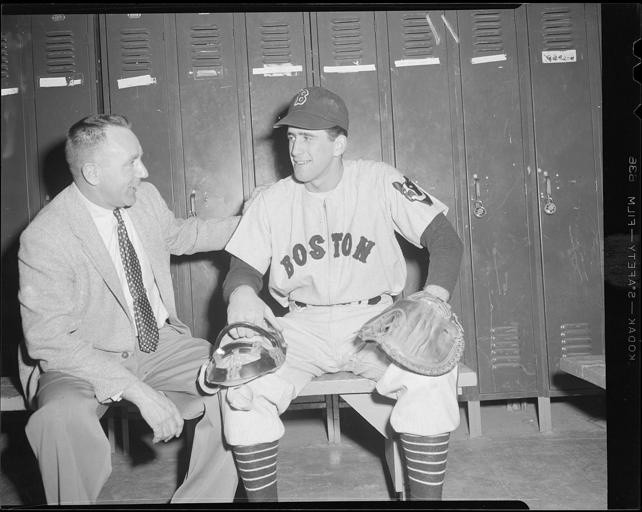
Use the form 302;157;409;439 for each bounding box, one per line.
114;208;160;354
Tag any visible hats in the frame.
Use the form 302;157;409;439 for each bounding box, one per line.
272;86;349;133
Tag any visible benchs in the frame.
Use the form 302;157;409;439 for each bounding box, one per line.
0;361;480;443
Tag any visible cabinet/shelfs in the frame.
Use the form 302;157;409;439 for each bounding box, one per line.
31;14;97;212
103;15;180;334
457;7;539;395
175;14;246;338
1;15;31;376
386;9;468;395
315;12;382;165
524;1;608;395
245;13;308;187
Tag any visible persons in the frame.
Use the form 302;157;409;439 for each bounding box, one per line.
198;86;466;505
17;113;275;505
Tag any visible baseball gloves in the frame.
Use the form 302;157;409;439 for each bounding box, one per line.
358;289;466;376
